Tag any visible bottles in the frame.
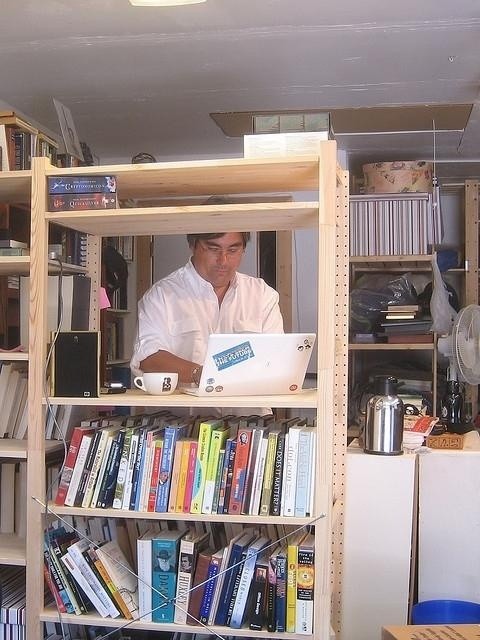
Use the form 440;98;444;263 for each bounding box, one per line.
440;380;466;434
363;373;406;456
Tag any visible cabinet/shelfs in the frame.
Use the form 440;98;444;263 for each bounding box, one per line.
349;253;438;436
0;111;350;640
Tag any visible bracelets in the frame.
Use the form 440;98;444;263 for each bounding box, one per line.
191;366;203;386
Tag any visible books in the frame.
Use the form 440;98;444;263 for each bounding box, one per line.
378;304;434;343
0;109;120;265
403;414;439;437
106;318;123;361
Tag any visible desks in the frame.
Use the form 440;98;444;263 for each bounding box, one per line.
346;437;479;639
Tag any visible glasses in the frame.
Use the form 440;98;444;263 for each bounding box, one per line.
195;238;244;256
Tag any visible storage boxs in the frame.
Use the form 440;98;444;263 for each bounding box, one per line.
346;157;445;257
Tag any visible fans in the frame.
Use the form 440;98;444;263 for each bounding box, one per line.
439;303;480;424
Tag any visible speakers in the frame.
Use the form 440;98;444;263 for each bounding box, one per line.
52;330;99;396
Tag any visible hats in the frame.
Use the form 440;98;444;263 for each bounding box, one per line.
156;550;171;558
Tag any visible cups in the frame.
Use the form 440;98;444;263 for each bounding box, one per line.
133;372;179;396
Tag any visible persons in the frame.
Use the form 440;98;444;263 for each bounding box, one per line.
130;196;277;418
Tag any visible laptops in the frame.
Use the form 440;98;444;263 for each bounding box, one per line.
178;331;317;398
